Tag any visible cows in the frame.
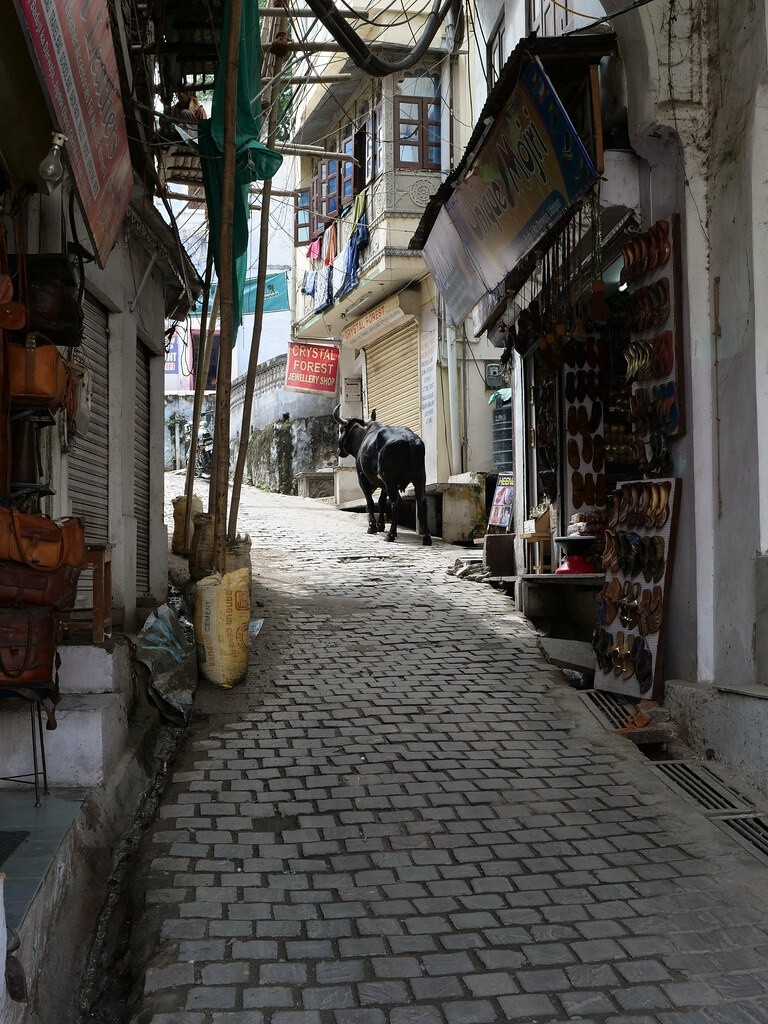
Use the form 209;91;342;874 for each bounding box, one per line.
332;403;433;546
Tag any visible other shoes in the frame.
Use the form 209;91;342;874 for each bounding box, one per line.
596;577;621;626
608;218;682;528
592;628;613;673
565;336;607;509
602;529;665;583
538;376;556;503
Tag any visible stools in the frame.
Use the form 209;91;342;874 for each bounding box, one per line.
55;541;116;643
519;532;551;573
0;690;48;809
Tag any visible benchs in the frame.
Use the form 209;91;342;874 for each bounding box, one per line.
296;471;334;497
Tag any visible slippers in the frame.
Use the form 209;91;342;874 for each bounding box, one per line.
637;586;663;636
609;631;635;679
613;581;641;630
630;636;652;693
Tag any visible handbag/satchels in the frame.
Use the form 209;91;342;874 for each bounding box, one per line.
0;506;87;570
0;560;81;612
7;342;66;406
0;301;26;330
16;284;82;347
0;273;14;304
0;607;54;685
